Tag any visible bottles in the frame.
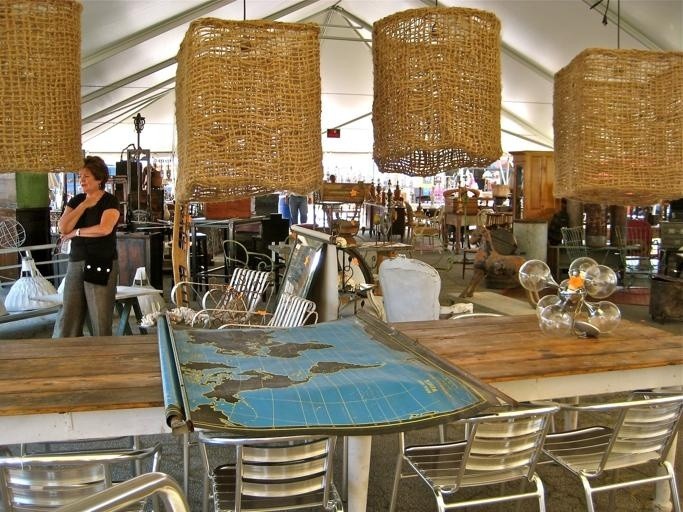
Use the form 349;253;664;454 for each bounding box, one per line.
428;209;431;217
163;205;170;220
126;194;135;230
146;195;153;221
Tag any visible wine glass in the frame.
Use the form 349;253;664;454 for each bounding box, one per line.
380;213;391;246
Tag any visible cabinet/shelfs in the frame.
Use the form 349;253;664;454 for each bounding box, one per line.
510;150;563;222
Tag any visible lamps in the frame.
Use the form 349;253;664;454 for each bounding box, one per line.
552;1;682;204
175;1;326;201
369;0;503;177
1;0;85;174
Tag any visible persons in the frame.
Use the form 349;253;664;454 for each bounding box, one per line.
51;154;123;337
327;174;343;220
284;171;312;226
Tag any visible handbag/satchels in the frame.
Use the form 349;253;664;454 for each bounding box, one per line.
82;253;112;285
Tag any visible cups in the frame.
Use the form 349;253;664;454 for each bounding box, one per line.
422;209;427;213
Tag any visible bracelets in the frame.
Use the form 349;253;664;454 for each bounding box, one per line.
75;227;81;237
